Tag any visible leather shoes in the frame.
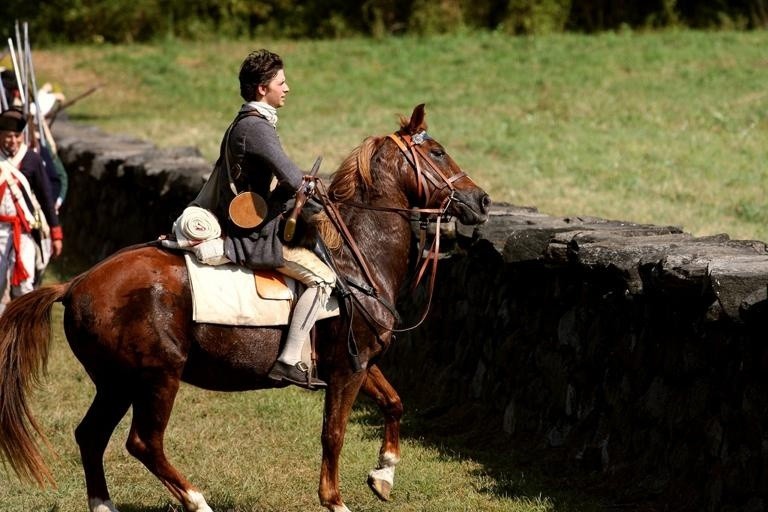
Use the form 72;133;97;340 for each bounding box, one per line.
269;360;327;386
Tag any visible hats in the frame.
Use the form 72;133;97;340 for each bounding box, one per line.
1;108;29;133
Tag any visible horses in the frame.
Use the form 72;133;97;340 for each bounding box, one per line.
1;102;492;512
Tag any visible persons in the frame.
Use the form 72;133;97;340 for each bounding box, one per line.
216;49;338;390
0;69;70;312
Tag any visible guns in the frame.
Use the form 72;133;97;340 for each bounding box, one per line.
43;87;96;120
283;156;324;242
0;20;46;157
